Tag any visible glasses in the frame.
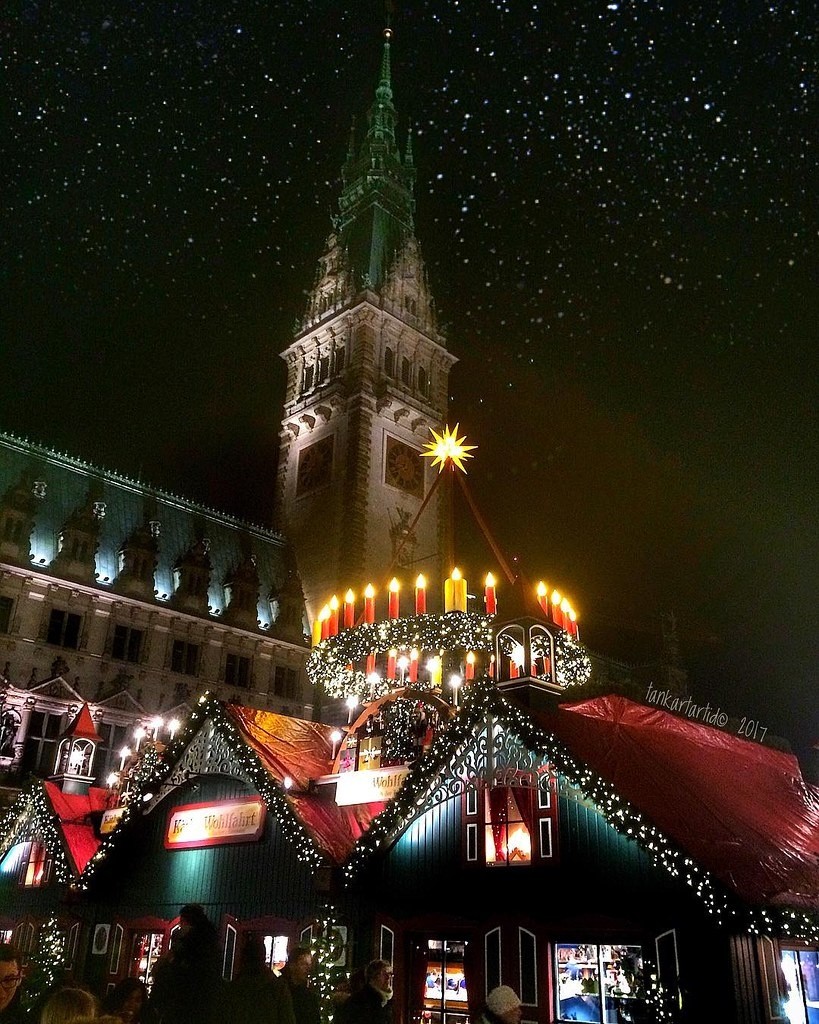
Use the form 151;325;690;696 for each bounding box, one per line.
0;970;21;989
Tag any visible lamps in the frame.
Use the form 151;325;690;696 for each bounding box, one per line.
489;655;496;679
321;604;329;639
536;581;548;617
444;567;467;613
572;607;577;637
510;659;519;679
485;571;497;615
389;577;399;619
312;611;321;648
329;648;418;762
566;606;572;634
432;649;444;686
329;594;339;636
365;582;375;622
542;655;550;673
414;574;427;615
551;589;563;627
561;598;567;631
450;650;476;707
344;588;354;629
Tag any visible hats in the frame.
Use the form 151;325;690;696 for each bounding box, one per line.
487;986;522;1015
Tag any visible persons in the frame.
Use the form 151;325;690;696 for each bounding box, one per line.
472;984;523;1024
0;904;323;1024
330;959;394;1024
